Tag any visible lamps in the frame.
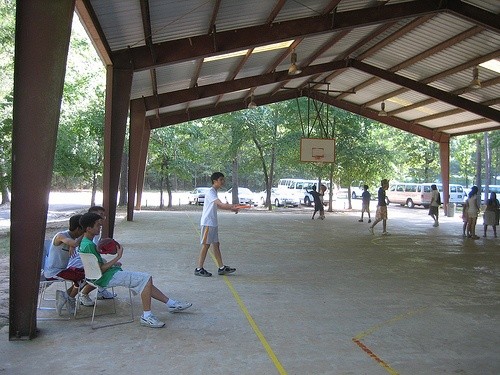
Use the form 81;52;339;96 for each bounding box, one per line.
288;48;303;75
469;65;482;89
248;93;258;109
378;100;388;117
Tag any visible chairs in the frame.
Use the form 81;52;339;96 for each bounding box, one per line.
74;252;135;329
36;239;80;320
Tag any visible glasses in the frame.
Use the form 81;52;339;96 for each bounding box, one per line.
99;212;106;220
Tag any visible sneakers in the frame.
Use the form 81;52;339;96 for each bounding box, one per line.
140;314;165;327
368;226;374;235
194;267;212;277
95;289;117;299
64;291;76;314
168;302;192;313
218;266;236;275
76;292;94;306
382;231;392;236
55;290;67;316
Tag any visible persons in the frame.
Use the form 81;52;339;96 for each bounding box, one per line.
306;185;324;220
462;199;469;237
467;186;480;240
428;185;439;227
483;199;498;238
75;206;122;306
368;179;391;236
358;185;371;223
490;192;500;225
194;172;237;278
44;214;87;316
79;213;192;328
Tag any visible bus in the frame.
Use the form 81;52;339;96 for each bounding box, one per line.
278;179;338;207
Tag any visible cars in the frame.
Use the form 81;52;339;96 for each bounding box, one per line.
260;188;300;207
224;188;259;207
188;188;211;205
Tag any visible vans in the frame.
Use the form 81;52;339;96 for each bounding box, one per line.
385;184;439;208
437;185;469;206
351;180;376;199
480;185;500;200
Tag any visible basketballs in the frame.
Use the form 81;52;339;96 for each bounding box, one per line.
95;237;121;255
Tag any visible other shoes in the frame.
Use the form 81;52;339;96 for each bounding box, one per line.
368;219;371;223
358;220;363;222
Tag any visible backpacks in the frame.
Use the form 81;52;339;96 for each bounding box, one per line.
436;192;441;205
